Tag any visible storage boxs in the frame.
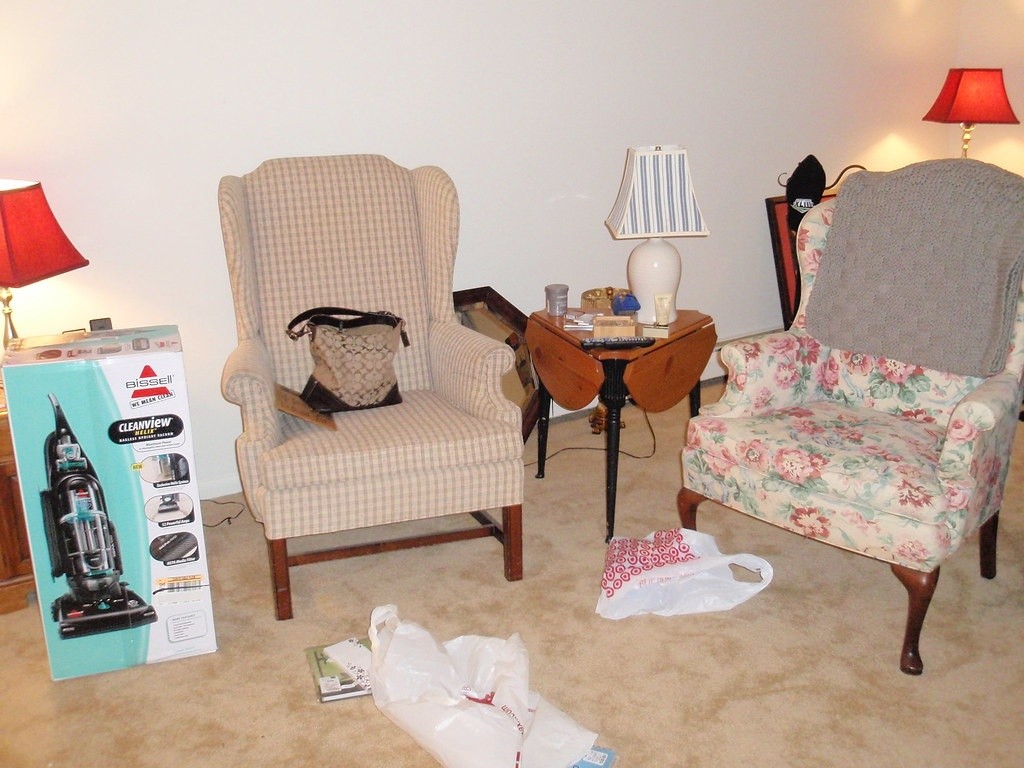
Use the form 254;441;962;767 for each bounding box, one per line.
1;325;218;682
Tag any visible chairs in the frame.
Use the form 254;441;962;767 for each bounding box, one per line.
217;153;527;622
676;157;1024;674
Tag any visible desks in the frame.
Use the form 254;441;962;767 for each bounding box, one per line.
524;307;717;545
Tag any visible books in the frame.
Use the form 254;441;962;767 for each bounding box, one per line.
305;637;375;703
571;745;618;768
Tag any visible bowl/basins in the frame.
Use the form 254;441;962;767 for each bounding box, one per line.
582;288;629;310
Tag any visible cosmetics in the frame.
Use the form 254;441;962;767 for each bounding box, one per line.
654;293;674;327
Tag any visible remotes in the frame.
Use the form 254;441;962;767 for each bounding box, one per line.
581;336;655;351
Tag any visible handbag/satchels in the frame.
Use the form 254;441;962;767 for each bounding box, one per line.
594;527;775;621
286;305;411;413
367;603;541;768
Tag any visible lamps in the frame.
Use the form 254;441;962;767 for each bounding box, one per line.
0;179;90;390
921;68;1020;159
603;144;709;326
